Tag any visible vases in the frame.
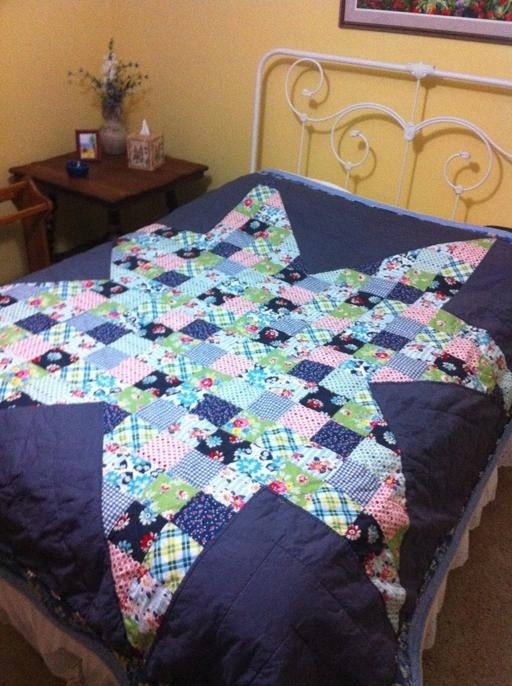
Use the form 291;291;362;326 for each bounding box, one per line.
100;119;127;157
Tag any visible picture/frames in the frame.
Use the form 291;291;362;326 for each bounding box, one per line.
337;0;512;45
75;129;102;162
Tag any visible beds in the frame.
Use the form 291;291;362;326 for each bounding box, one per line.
0;51;512;686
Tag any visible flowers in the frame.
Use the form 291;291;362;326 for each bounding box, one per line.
68;39;148;120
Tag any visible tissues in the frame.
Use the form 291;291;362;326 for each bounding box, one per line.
126;116;165;171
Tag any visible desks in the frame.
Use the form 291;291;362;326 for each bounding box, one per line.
9;144;209;258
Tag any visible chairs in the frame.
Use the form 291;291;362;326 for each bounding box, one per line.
0;176;54;287
